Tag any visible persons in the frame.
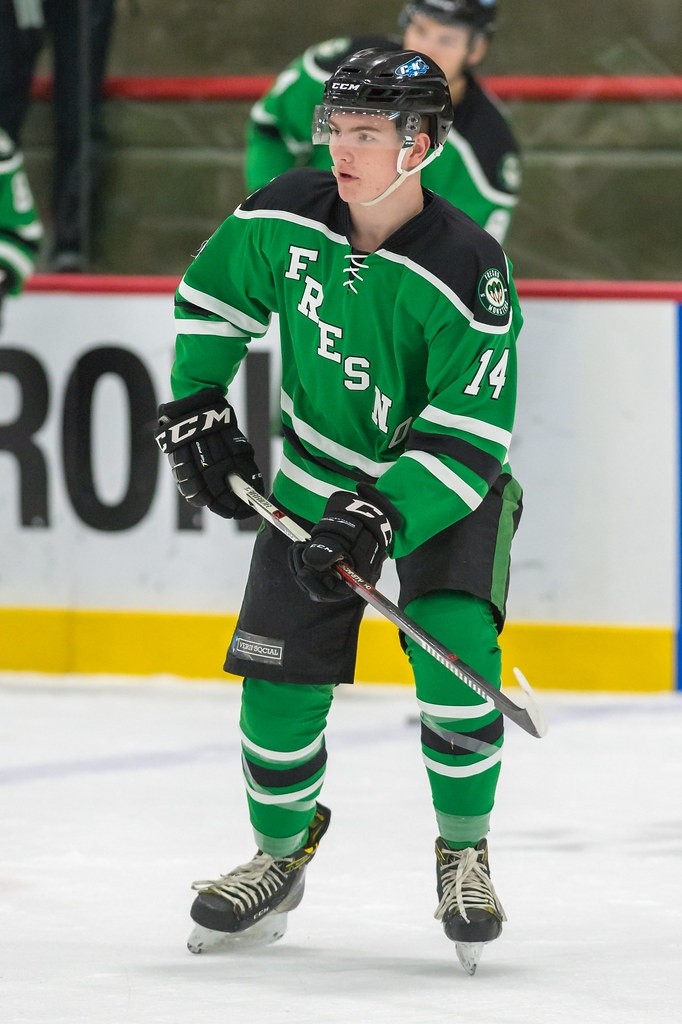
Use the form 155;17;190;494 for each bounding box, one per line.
0;0;116;274
244;0;523;246
154;48;524;974
1;130;44;295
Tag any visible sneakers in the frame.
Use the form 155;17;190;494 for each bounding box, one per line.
185;813;330;957
433;831;509;975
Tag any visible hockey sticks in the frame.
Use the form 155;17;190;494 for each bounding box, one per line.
229;468;545;740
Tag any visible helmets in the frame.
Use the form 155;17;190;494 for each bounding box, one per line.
407;2;499;35
319;46;453;122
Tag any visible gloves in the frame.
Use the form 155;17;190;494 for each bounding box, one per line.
288;488;396;606
156;387;262;518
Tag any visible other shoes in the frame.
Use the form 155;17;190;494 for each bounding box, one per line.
52;242;89;270
